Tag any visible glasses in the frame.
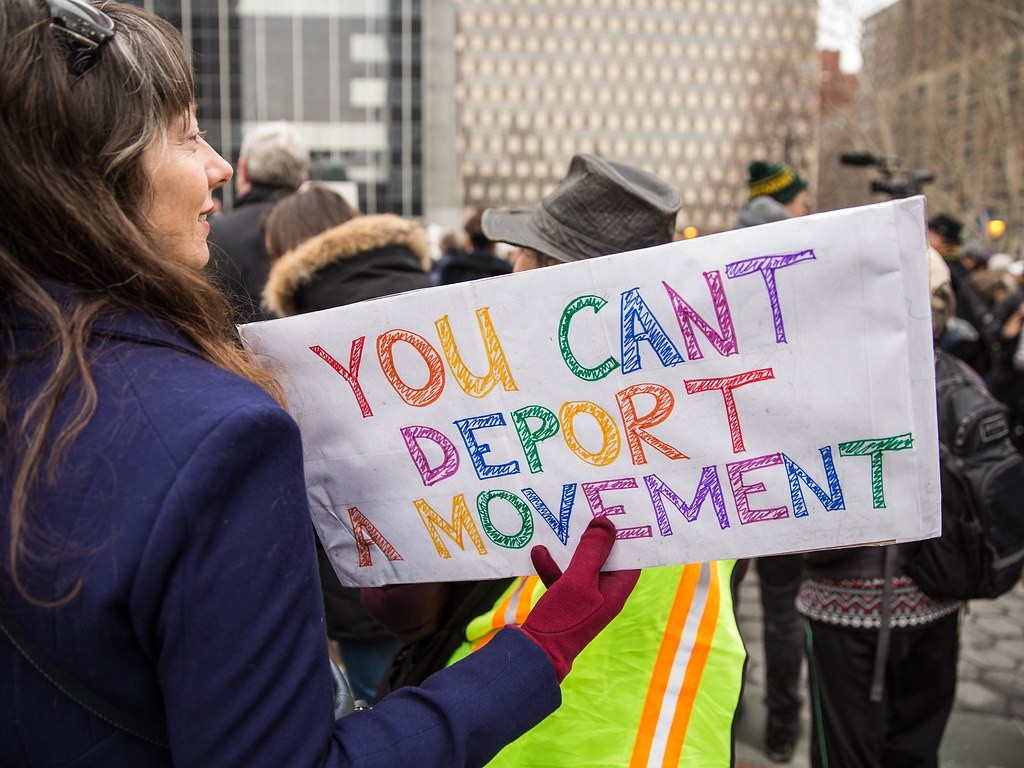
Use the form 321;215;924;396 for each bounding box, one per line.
32;0;116;109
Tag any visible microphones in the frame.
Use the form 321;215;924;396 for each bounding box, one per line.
841;152;883;165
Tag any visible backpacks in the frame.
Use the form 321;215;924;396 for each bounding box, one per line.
902;347;1024;603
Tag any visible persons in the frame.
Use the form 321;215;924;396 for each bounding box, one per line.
0;0;643;768
195;122;1024;768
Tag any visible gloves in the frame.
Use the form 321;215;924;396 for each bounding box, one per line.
503;516;642;685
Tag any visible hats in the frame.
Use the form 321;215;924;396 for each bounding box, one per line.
928;215;962;245
748;159;809;204
479;153;683;263
